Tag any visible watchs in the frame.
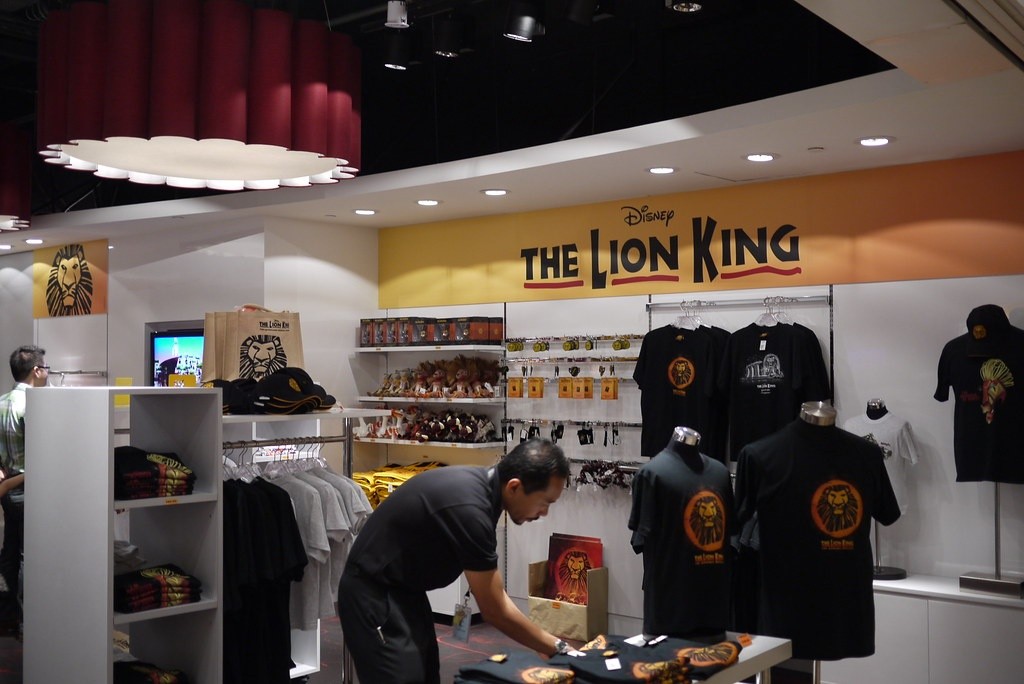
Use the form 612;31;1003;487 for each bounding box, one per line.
549;641;567;658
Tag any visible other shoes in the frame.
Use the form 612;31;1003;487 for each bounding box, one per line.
6;622;23;643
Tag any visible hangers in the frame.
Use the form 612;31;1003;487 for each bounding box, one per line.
754;294;798;327
222;435;328;480
670;300;715;330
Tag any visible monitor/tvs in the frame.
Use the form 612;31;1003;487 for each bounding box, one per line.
148;329;204;387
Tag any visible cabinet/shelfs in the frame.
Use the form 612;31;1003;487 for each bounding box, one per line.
354;345;505;451
19;386;224;684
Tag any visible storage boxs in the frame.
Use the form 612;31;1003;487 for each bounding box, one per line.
358;316;506;348
526;560;609;643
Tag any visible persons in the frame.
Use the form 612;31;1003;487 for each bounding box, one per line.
338;438;577;684
0;347;47;625
627;426;741;646
843;398;920;517
934;304;1024;484
736;401;901;662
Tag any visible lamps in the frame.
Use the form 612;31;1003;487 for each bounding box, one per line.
384;1;411;30
561;0;596;27
672;0;703;14
433;17;465;59
35;0;366;194
499;0;541;46
382;30;412;71
0;121;33;237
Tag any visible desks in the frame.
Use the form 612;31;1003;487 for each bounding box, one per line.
573;630;793;684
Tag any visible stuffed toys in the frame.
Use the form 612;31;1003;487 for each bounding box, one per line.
354;406;495;442
368;354;499;397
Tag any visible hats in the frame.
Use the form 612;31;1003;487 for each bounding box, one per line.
965;304;1010;358
201;367;335;416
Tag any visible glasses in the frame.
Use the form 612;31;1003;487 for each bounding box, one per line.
33;363;50;373
